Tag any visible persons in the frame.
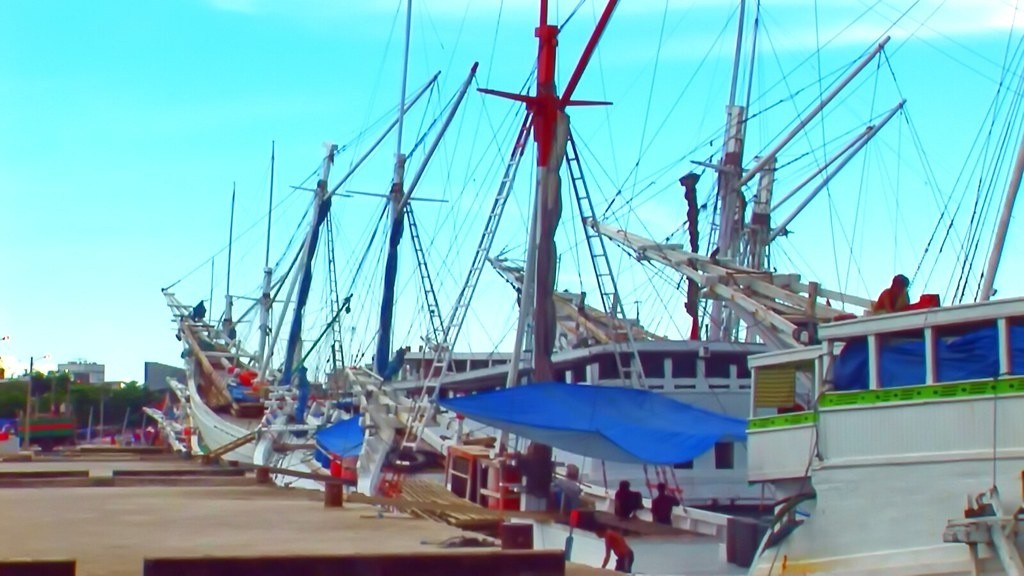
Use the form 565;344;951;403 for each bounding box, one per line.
593;523;634;573
873;274;910;315
650;482;680;527
614;480;642;519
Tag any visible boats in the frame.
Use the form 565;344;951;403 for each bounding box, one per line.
166;229;815;575
